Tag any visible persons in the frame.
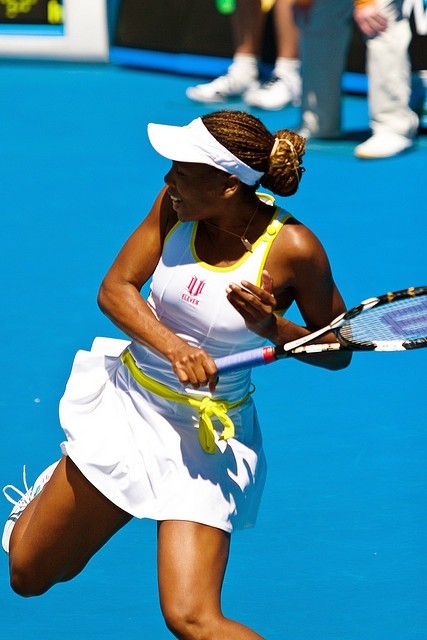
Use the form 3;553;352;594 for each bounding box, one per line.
2;110;351;640
185;0;312;111
353;0;427;160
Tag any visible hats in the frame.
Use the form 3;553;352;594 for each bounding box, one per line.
146;117;264;189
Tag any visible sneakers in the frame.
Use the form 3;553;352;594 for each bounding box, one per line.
3;458;73;559
183;73;263;106
247;81;301;111
355;126;416;160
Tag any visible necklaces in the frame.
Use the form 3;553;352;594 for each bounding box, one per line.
198;199;261;252
